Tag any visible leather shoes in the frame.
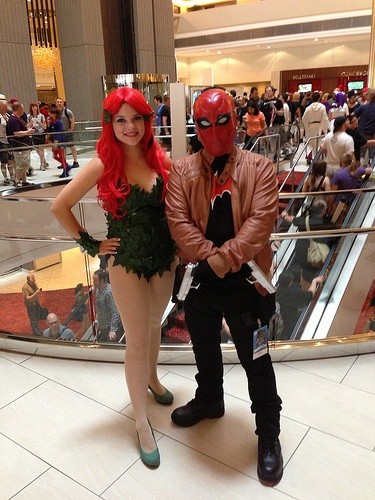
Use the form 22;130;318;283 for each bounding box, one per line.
171;389;225;427
256;434;283;482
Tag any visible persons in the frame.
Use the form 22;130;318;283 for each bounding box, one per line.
51;87;182;469
165;88;284;481
0;87;375;341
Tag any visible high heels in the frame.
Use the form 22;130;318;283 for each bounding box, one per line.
137;416;160;467
148;380;174;404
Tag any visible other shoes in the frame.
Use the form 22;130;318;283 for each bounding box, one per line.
40;163;49;170
71;161;79;168
60;165;71;178
15;182;35;186
57;163;67;169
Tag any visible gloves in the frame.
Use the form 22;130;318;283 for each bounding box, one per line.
225;262;253;284
191;259;222;287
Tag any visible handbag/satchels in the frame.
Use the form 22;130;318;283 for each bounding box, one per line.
306;242;330;266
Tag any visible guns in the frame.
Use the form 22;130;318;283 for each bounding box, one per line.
245;258;277;295
175;262;200;301
